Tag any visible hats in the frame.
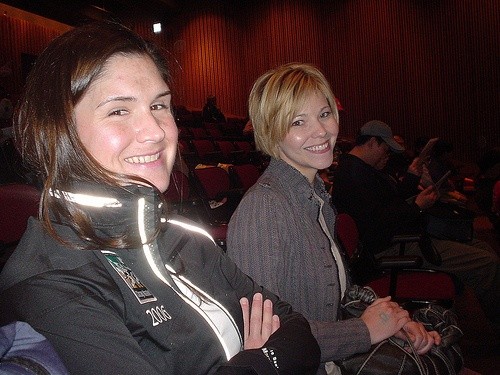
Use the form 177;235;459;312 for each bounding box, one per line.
360;119;404;154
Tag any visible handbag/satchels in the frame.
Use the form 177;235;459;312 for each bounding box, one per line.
334;285;465;375
428;198;476;243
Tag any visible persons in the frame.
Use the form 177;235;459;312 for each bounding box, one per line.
203;95;225;123
0;18;324;375
331;120;500;321
225;62;442;375
242;119;254;136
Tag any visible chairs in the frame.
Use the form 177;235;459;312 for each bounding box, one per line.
0;104;500;375
